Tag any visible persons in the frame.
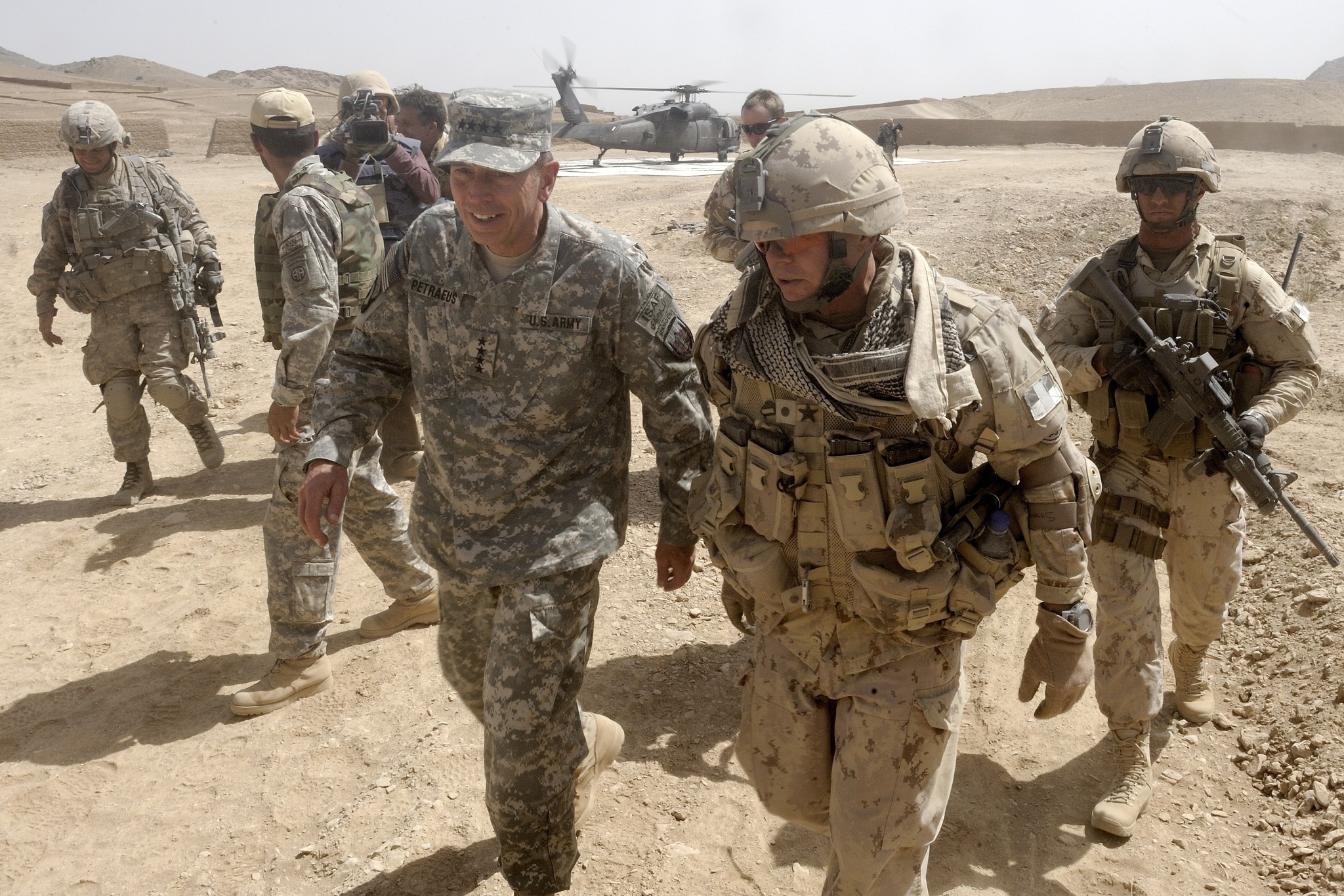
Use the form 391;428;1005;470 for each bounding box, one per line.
24;99;227;508
304;72;439;480
1038;119;1325;833
696;116;1103;893
877;117;904;168
289;79;713;896
217;86;443;718
388;84;453;200
704;88;787;275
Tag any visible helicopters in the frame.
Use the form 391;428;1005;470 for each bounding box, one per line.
535;35;743;168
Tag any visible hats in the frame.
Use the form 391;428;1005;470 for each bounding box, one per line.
432;86;554;173
249;87;316;129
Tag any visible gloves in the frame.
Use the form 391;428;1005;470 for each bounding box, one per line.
1103;339;1172;401
1203;419;1266;477
194;262;225;298
721;578;757;638
1017;601;1094;720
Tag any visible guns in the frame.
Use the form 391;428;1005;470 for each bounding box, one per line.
99;201;164;238
1068;256;1342;568
159;203;226;399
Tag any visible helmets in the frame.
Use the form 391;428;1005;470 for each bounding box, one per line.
336;69;400;121
1115;114;1222;193
58;99;133;149
734;112;909;243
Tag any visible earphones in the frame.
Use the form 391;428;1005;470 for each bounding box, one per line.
1189;221;1215;299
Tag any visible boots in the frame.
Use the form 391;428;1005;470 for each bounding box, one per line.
111;456;155;506
230;651;334;716
1089;728;1153;838
570;711;625;832
184;415;224;470
1167;637;1215;722
359;583;441;638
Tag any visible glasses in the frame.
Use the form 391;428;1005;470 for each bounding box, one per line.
742;117;789;135
1125;175;1200;196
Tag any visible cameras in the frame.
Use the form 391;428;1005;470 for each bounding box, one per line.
326;87;389;147
